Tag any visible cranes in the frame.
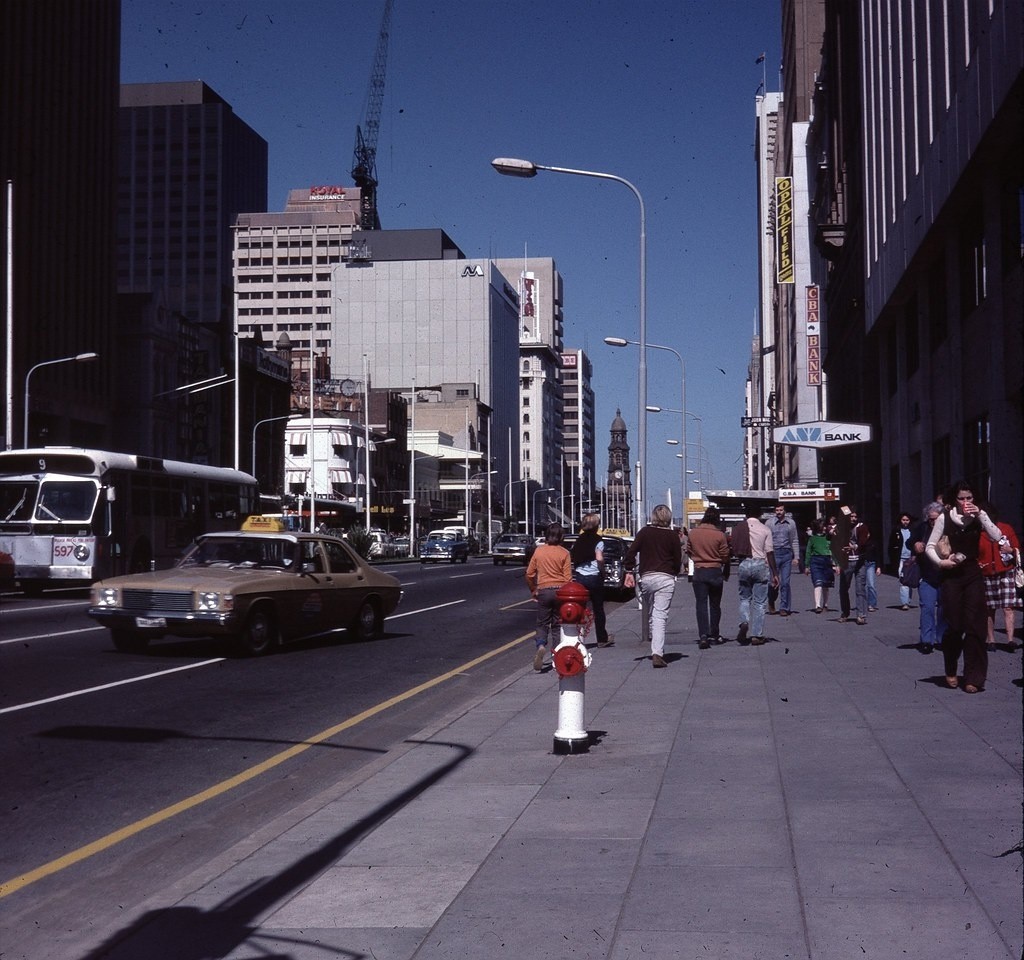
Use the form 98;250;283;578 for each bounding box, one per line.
350;0;396;231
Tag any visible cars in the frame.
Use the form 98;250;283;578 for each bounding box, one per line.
0;552;16;594
367;532;417;561
444;526;480;556
419;530;470;564
492;534;536;566
88;532;404;658
534;537;638;602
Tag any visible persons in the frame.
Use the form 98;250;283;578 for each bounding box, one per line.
925;481;1002;693
525;523;573;671
624;504;682;668
318;518;328;535
687;507;729;649
805;508;881;624
571;513;614;648
765;503;799;616
388;530;407;537
888;513;918;610
731;503;780;645
905;489;1024;654
676;526;689;574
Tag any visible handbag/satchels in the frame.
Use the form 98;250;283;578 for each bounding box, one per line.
899;559;920;588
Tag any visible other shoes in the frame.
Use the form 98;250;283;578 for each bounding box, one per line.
768;604;775;613
946;676;958;687
736;623;765;645
698;635;725;649
653;654;667;667
1015;548;1024;588
533;646;547;670
779;609;788;616
965;685;977;692
839;616;849;623
857;615;867;624
598;634;614;647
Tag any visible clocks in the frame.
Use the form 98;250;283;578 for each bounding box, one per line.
340;379;355;396
615;471;623;479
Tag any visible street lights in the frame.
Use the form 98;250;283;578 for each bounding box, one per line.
666;440;713;490
253;415;303;477
355;438;396;512
646;405;702;491
408;454;445;498
491;158;645;611
604;337;686;532
503;480;592;538
470;471;498;526
24;353;99;449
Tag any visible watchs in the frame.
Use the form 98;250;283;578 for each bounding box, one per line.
978;506;982;515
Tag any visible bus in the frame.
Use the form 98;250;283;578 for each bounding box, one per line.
0;444;260;601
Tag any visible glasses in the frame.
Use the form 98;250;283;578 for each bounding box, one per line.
957;497;971;500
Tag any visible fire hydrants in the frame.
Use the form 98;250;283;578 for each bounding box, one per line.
550;582;593;740
